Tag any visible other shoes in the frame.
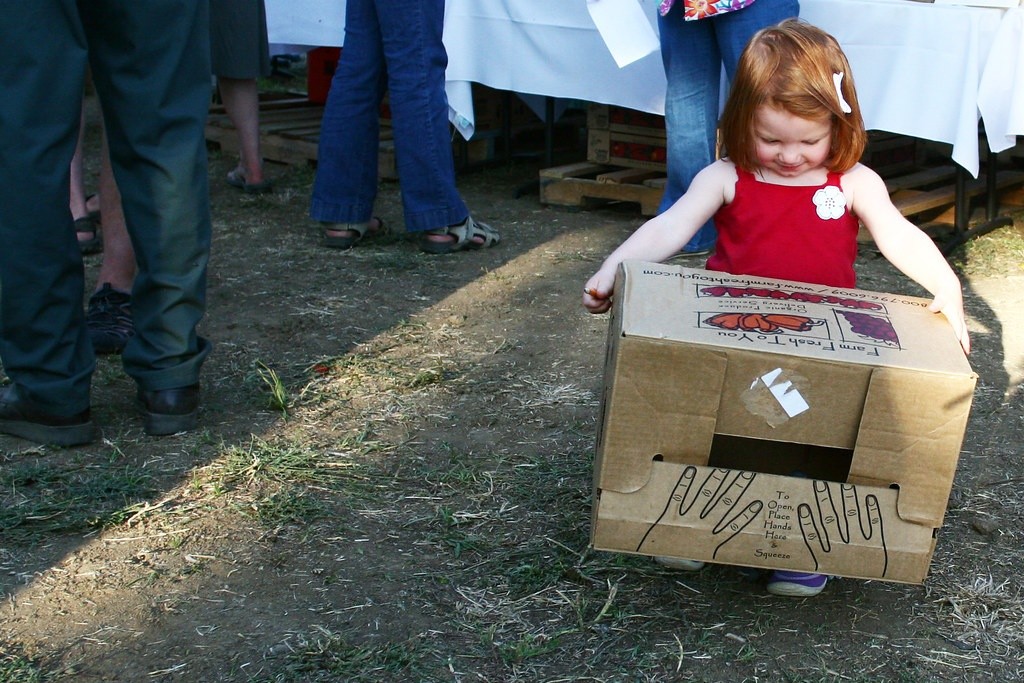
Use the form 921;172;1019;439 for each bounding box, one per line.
652;556;705;572
664;249;709;261
766;570;828;597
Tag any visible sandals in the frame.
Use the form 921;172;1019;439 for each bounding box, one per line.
317;217;389;248
83;195;102;226
84;283;137;356
417;217;501;250
74;215;102;256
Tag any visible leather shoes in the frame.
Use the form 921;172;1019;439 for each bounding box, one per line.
137;380;201;432
0;379;97;446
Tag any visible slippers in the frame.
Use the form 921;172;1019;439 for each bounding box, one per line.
224;164;274;194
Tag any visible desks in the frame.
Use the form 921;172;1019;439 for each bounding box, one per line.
217;0;1024;258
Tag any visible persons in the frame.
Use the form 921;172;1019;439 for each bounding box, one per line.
660;0;801;253
583;19;972;594
0;0;268;448
310;0;503;251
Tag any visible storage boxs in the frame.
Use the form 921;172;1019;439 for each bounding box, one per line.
586;130;667;172
590;260;978;585
307;47;342;105
586;101;667;138
